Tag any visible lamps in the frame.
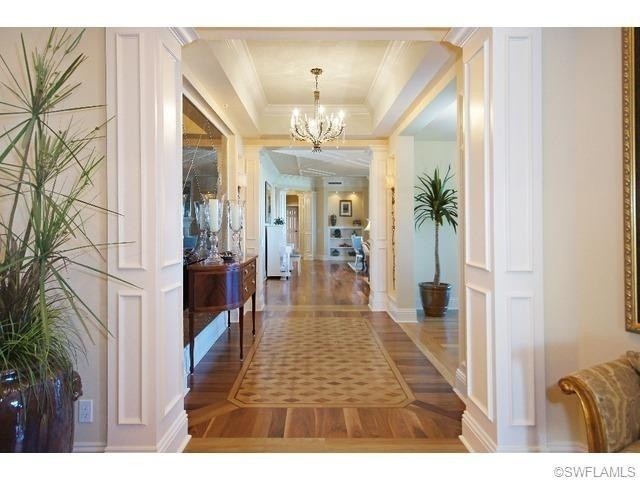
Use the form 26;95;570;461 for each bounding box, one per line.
289;67;348;153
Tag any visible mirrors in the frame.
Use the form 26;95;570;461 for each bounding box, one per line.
183;94;229;266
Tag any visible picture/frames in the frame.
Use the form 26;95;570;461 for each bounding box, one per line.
339;199;352;218
264;181;273;224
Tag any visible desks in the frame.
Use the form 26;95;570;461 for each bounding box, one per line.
188;253;259;375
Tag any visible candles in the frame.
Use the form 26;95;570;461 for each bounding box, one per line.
198;205;205;230
232;204;240;231
210;200;220;231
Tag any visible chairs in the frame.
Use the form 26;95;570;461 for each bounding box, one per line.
352;235;366;271
557;350;639;453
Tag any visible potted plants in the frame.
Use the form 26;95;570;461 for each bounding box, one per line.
2;28;143;452
411;164;459;319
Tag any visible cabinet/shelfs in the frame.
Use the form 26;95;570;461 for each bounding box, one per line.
266;225;291;281
329;225;362;262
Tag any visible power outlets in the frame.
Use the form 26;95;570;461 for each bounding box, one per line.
77;398;96;424
